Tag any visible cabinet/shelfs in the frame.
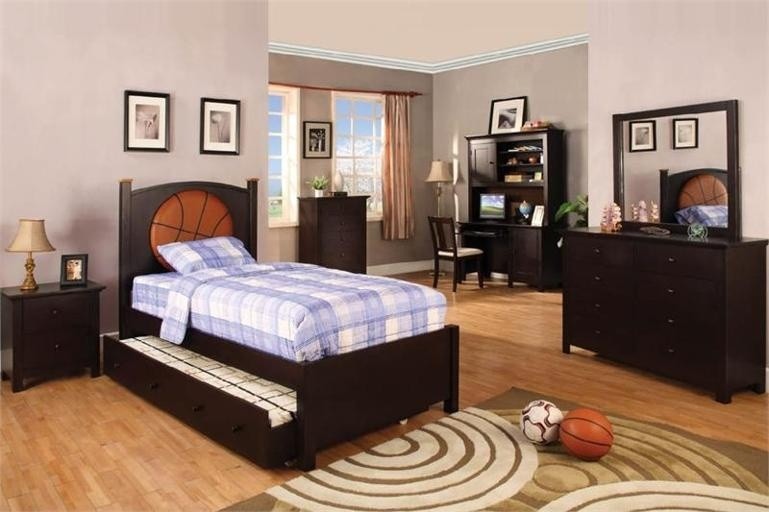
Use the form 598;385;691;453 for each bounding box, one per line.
298;195;370;274
562;226;769;404
464;129;565;292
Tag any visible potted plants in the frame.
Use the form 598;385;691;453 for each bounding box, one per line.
305;174;328;197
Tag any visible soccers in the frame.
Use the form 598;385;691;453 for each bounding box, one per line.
519;399;564;447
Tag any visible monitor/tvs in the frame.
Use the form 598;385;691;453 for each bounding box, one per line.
479;193;506;219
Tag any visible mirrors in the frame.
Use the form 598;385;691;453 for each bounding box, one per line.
613;100;741;239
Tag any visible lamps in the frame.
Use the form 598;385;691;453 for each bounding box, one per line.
6;218;55;291
425;161;455;279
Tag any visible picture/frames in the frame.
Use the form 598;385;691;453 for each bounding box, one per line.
124;89;171;153
673;117;699;147
200;97;241;154
61;256;86;286
304;121;332;159
488;96;527;134
629;122;655;153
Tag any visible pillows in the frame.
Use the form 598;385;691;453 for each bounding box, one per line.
157;235;258;275
676;204;728;228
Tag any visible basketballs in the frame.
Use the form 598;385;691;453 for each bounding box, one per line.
150;190;233;271
677;175;727;210
559;407;614;463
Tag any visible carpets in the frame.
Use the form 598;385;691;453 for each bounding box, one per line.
219;386;769;512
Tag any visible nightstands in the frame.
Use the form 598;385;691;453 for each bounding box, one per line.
0;280;106;390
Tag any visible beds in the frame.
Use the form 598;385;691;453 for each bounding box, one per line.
104;177;461;470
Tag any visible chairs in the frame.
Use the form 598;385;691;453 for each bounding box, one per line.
425;216;483;291
